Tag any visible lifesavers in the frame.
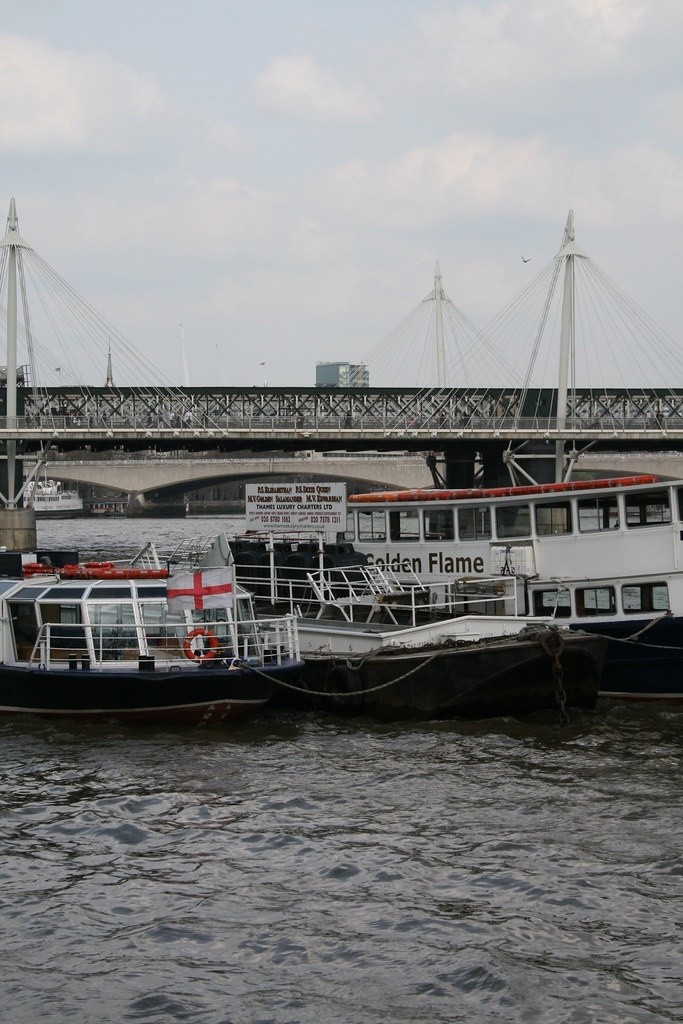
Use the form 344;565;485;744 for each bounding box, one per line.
183;629;219;664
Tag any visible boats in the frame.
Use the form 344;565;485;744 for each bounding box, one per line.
80;482;611;719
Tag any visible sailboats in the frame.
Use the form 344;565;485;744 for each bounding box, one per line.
348;208;683;699
0;197;306;720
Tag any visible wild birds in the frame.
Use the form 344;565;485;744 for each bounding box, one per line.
522;256;531;263
